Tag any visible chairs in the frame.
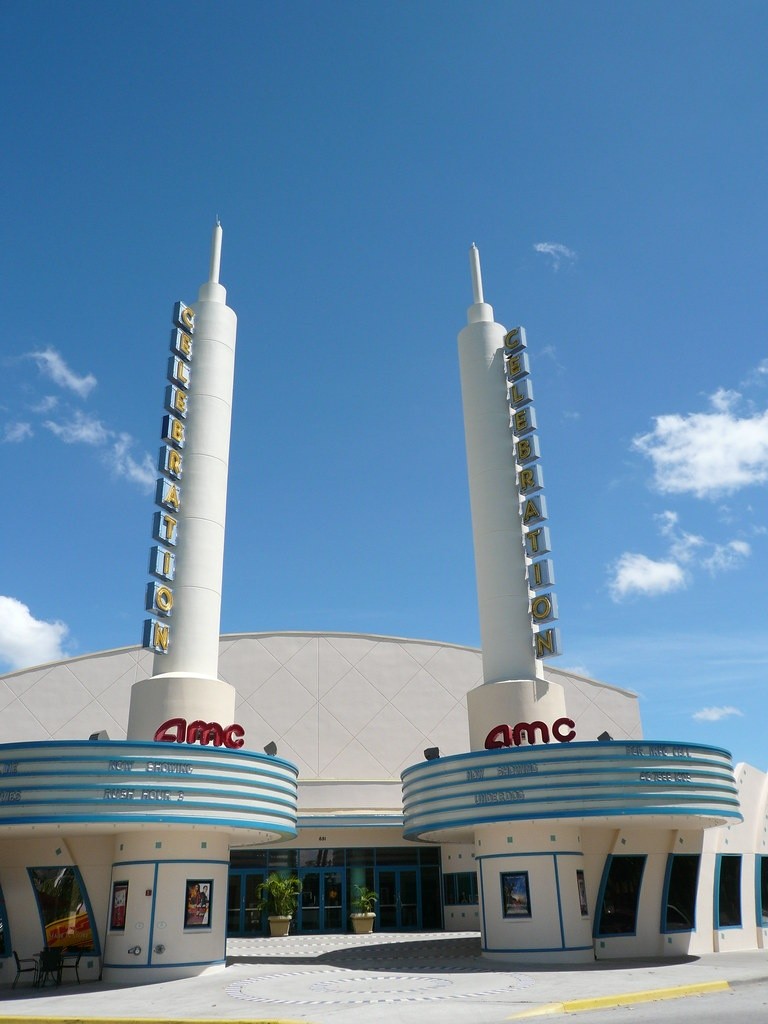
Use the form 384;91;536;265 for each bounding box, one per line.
11;946;85;991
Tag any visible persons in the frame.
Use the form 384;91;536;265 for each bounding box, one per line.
189;884;201;906
200;886;209;906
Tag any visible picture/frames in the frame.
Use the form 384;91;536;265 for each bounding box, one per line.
184;879;214;930
108;879;129;933
501;871;532;920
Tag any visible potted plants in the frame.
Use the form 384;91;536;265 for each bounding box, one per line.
256;871;306;938
350;885;378;934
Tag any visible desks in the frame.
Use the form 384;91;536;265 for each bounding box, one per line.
32;953;65;987
